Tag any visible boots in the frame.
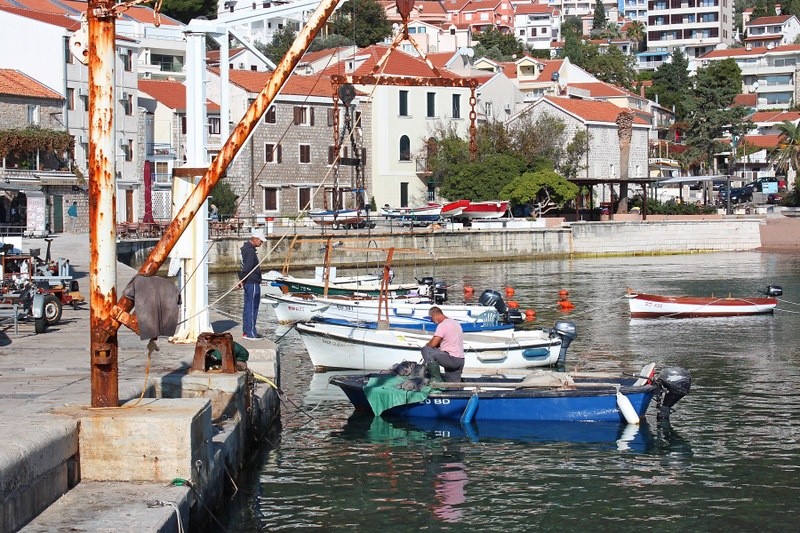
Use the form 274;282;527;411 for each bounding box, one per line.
426;361;443;386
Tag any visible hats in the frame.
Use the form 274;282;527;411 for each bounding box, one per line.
252;229;267;242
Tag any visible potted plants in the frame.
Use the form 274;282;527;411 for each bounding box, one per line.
370;196;378;220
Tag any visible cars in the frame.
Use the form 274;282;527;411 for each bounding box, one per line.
722;188;753;203
712;180;728;191
745;177;787;193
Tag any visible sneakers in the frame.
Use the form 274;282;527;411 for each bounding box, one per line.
242;332;265;340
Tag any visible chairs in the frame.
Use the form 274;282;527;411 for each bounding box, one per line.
473;311;499;328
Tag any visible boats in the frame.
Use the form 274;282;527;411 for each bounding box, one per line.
294;319;577;371
397;200;470;217
428;201;509;219
328;361;692;423
258;270;525;332
628;285;783;321
380;203;441;225
308;208;368;224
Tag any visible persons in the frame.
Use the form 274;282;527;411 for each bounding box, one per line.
421;307;465;391
67;202;78;234
524;203;536;218
696;200;702;210
400;464;468;530
238;229;265;340
670;195;681;206
383;203;390;220
209;204;219;222
